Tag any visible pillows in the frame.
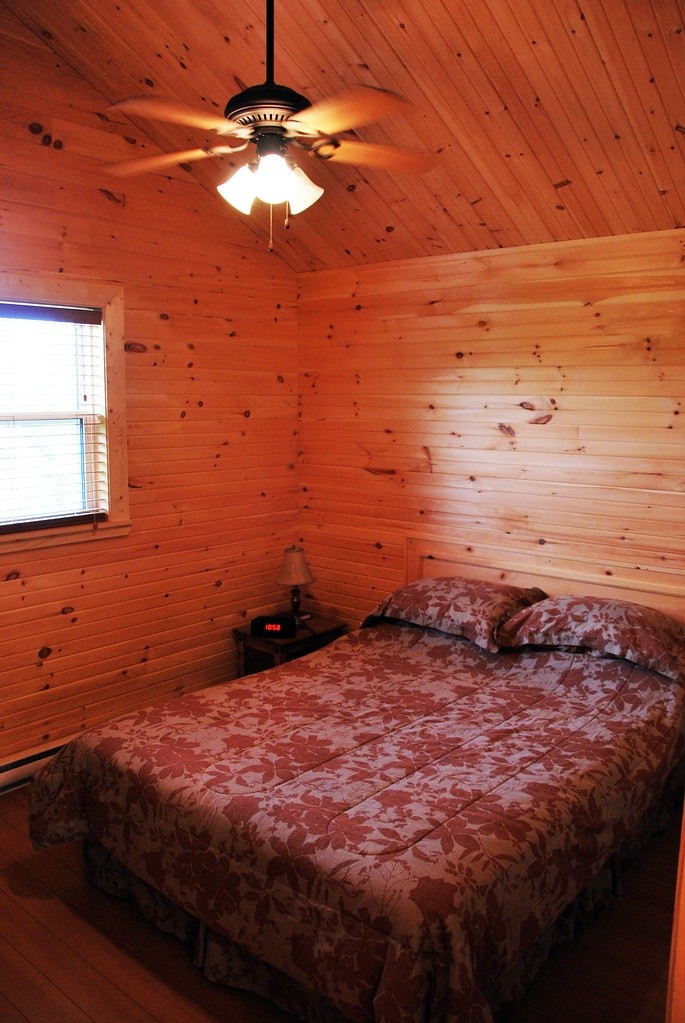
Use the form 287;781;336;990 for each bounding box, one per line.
494;587;685;685
359;577;531;655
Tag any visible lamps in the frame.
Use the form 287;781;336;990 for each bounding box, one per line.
217;139;325;253
274;543;317;621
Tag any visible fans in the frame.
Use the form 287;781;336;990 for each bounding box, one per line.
104;0;438;180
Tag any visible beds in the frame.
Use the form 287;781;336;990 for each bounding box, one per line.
27;538;685;1023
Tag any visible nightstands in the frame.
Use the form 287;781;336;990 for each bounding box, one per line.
233;612;348;678
